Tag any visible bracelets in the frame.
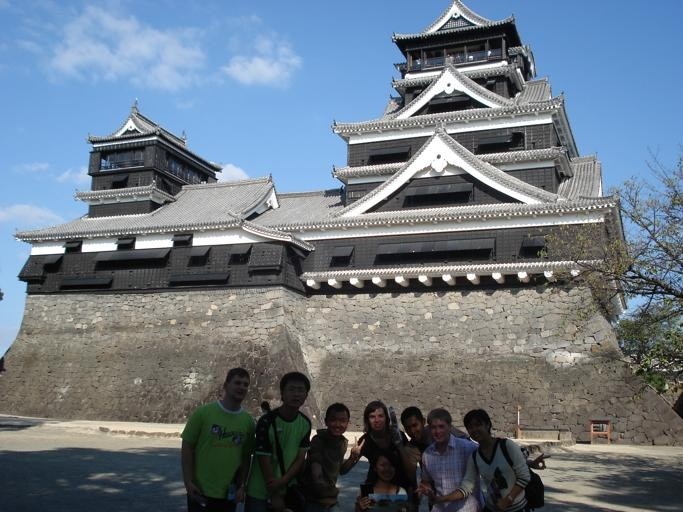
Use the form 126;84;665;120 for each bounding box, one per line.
507;494;514;502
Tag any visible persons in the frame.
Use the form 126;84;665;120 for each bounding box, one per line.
430;409;532;511
245;371;312;512
300;403;350;511
180;368;256;512
352;447;415;512
392;405;470;474
341;399;412;492
415;408;551;511
261;401;271;414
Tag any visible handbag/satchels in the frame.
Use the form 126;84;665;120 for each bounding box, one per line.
524;467;545;509
